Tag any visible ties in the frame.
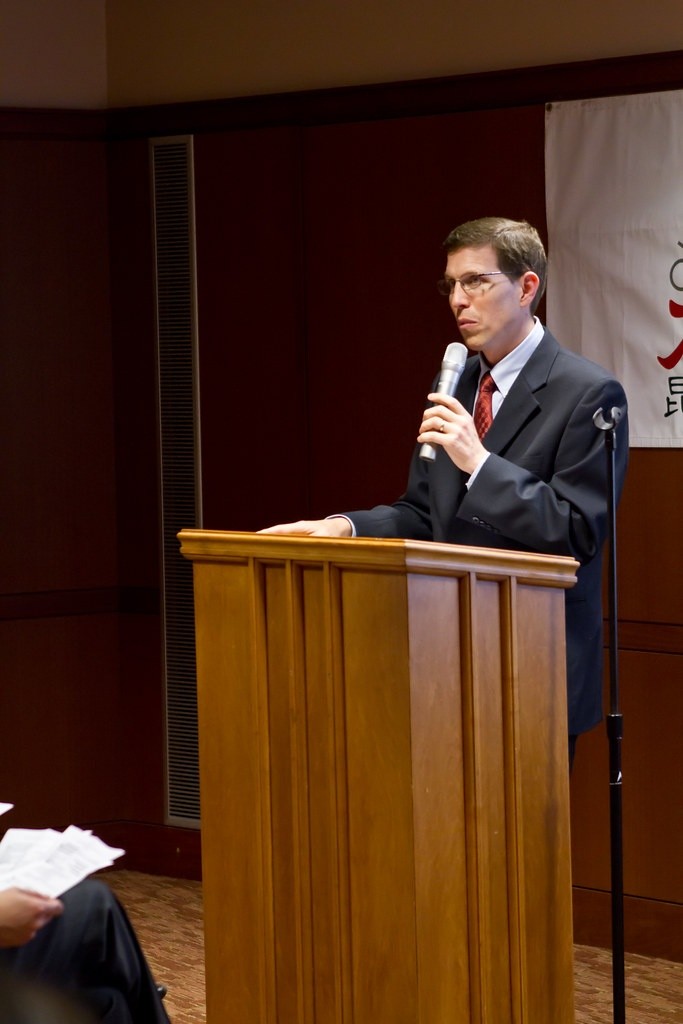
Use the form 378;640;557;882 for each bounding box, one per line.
465;370;498;443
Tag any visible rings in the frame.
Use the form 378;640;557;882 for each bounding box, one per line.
440;419;444;432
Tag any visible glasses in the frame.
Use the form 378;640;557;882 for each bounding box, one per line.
438;270;508;292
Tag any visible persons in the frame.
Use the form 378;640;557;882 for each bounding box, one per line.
256;216;630;777
0;803;170;1024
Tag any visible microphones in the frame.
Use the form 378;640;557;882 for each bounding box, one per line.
419;342;469;462
